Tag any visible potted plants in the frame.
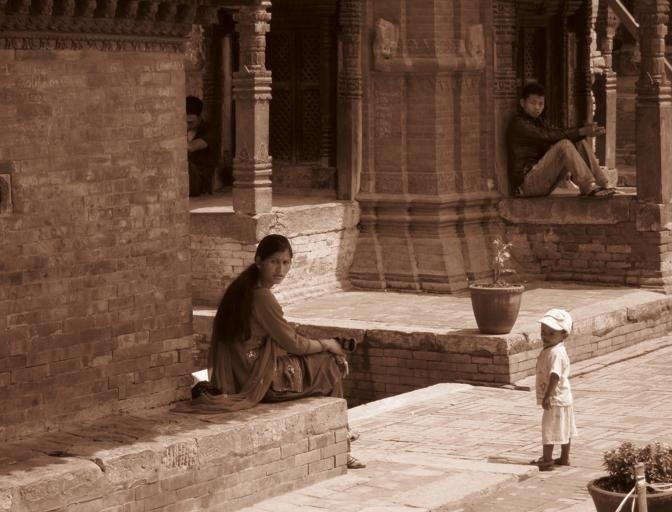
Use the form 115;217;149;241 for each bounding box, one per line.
587;443;672;512
467;239;526;336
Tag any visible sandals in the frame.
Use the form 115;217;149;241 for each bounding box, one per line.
347;454;366;468
531;457;569;471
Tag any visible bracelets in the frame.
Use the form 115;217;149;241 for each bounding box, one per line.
318;339;326;353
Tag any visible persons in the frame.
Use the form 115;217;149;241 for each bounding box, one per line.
186;95;220;196
192;234;365;468
507;81;618;199
531;308;575;471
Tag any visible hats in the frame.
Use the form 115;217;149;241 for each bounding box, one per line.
537;308;572;335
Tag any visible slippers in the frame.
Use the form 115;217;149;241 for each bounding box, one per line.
577;187;615;199
